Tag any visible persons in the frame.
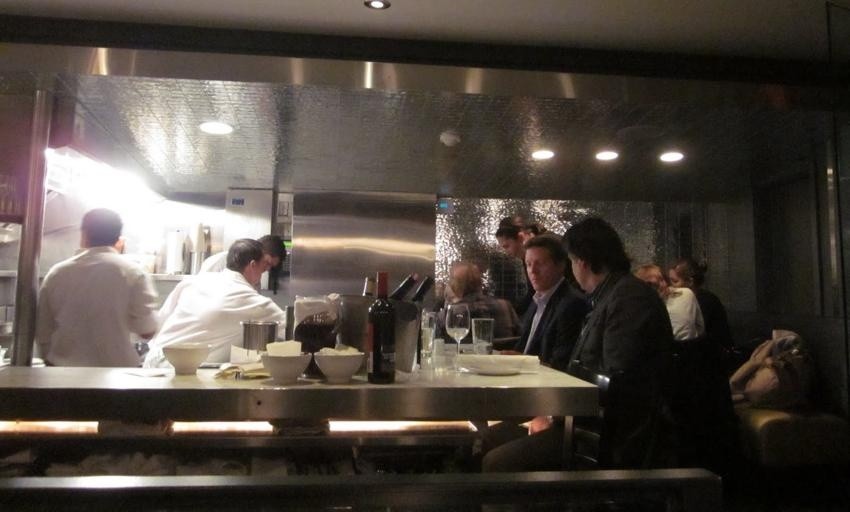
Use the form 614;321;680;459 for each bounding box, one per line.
199;234;284;291
430;215;756;508
140;239;286;369
36;207;162;368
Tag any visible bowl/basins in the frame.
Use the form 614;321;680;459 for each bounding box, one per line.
260;350;313;384
312;351;365;383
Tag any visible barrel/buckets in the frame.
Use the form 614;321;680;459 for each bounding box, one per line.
240;319;277;351
339;293;420;373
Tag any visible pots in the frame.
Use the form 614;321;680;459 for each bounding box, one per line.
161;342;213;375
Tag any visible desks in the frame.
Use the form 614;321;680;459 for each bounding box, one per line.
1;345;601;471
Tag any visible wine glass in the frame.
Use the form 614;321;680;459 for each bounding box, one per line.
445;302;470;373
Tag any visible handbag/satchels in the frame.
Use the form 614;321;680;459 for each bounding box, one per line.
729;329;815;410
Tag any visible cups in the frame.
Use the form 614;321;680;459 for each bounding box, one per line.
471;317;495;356
421;311;438;359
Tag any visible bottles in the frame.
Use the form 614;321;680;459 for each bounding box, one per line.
362;272;436;302
366;270;396;385
